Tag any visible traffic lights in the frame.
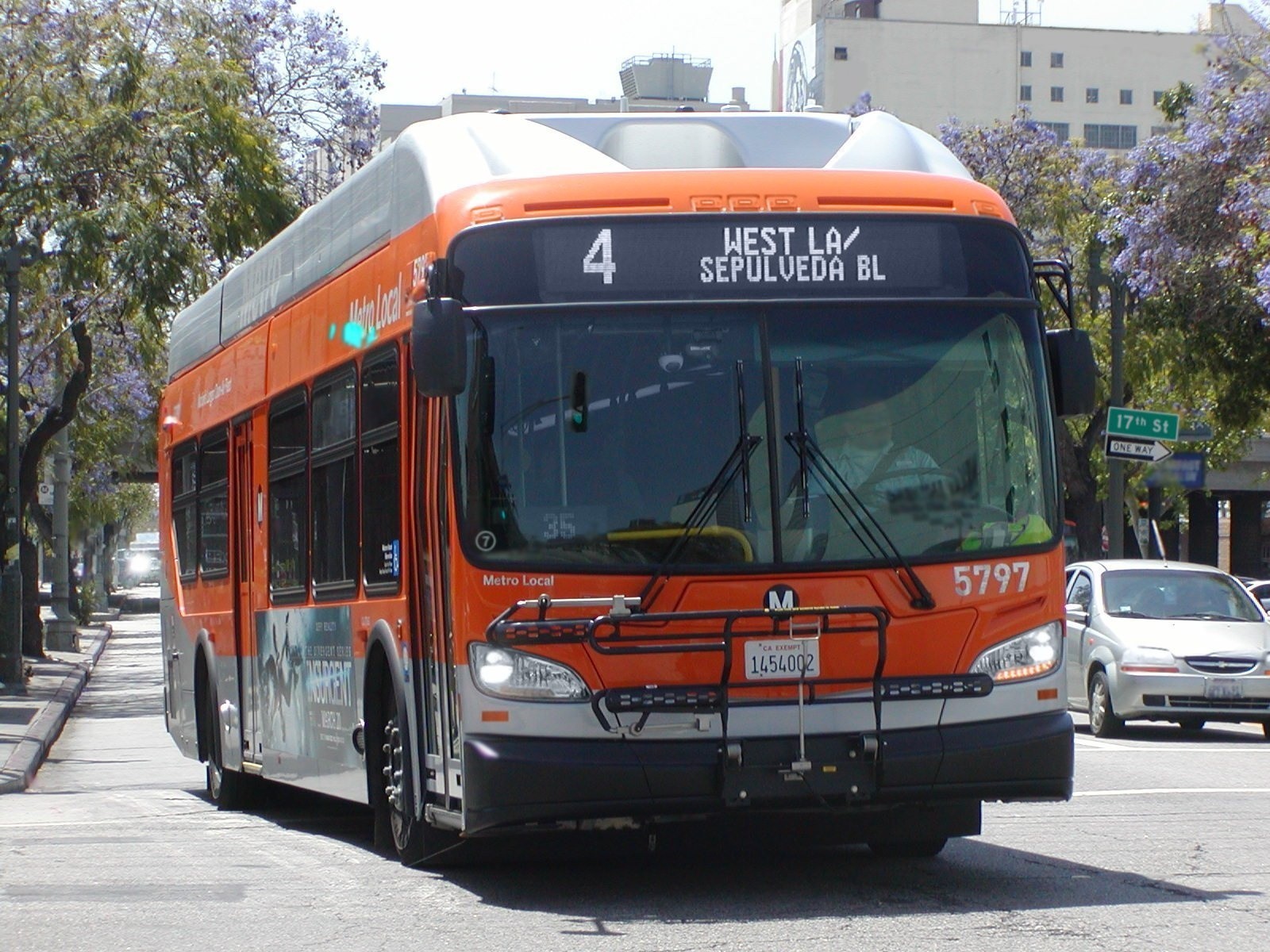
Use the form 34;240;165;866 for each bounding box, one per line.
573;370;589;437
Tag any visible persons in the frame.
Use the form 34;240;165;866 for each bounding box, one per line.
800;394;954;537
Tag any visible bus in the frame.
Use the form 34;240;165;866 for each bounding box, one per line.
155;106;1102;870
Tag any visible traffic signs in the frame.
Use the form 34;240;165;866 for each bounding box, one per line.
1104;435;1173;464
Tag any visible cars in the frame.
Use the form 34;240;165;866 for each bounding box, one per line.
129;557;160;587
1065;558;1270;742
1231;573;1270;618
